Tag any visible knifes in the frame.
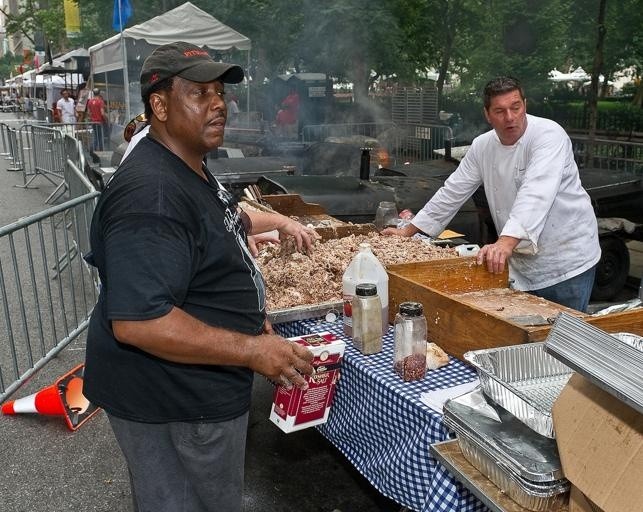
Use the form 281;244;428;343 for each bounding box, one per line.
503;315;564;326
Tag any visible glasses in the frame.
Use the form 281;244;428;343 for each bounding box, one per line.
124;113;148;142
216;187;252;233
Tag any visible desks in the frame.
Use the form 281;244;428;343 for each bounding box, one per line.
271;314;489;512
430;440;569;512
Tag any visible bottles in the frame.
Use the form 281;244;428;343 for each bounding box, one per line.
376;202;399;229
353;282;383;357
392;301;428;382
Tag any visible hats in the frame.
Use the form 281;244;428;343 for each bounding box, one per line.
141;41;244;95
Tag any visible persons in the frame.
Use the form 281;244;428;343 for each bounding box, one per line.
88;88;108;151
276;84;300;126
81;39;316;510
114;120;322;256
379;76;601;314
56;89;79;139
225;92;238;128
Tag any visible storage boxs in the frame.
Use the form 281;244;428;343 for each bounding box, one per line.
551;370;643;511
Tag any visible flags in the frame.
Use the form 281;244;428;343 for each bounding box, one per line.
47;44;52;65
112;0;132;32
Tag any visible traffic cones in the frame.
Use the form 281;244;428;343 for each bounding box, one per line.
1;363;102;433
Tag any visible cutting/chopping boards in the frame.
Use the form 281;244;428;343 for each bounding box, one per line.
446;286;592;328
